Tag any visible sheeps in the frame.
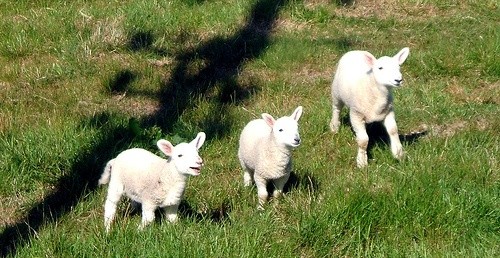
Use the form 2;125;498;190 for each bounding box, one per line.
329;47;409;168
98;131;206;236
238;106;304;211
